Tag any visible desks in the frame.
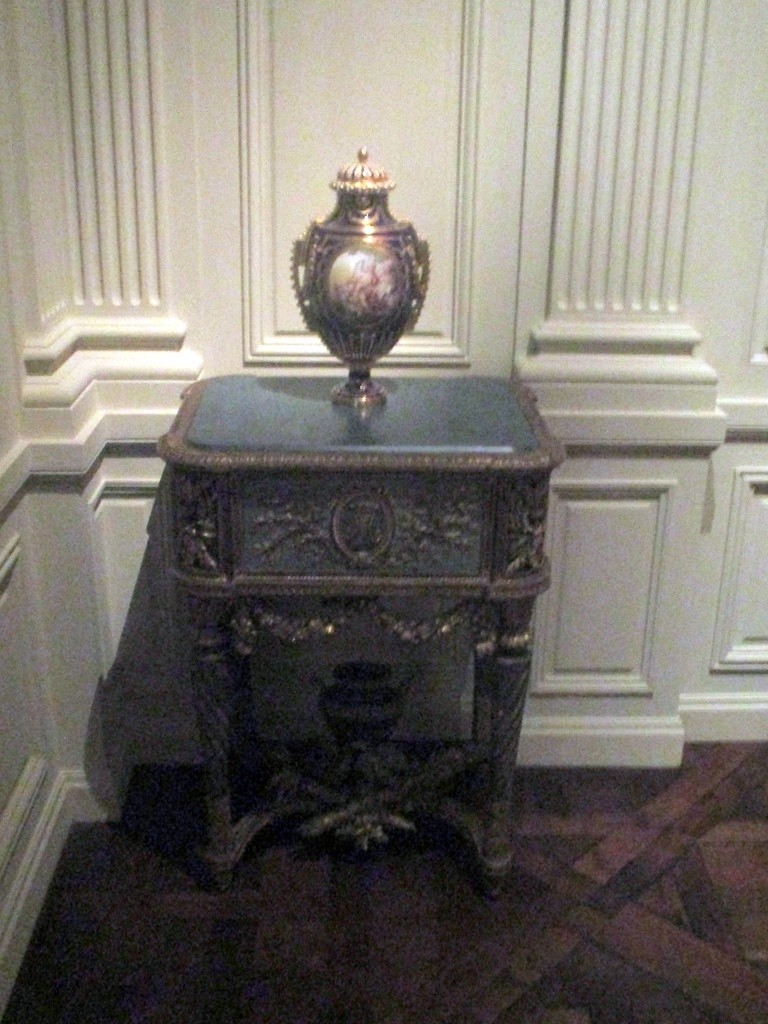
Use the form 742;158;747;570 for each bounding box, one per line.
152;375;567;900
